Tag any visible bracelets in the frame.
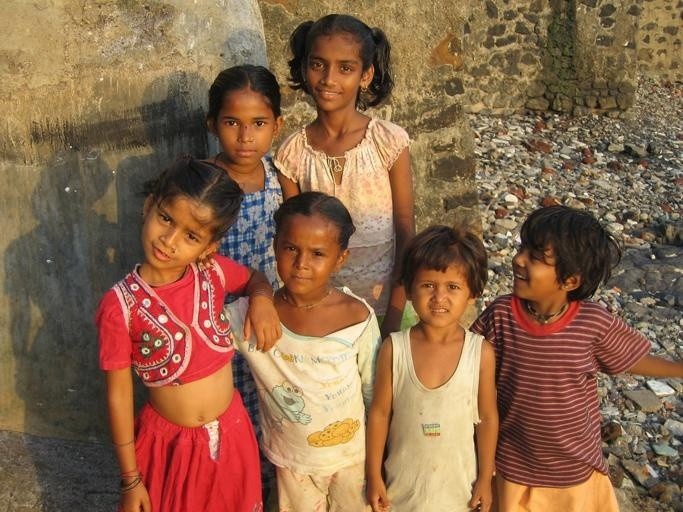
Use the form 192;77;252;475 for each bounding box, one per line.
111;439;143;493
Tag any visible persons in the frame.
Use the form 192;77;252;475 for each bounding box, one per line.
218;189;384;512
468;205;682;512
203;64;302;512
365;224;499;512
269;11;417;340
93;150;284;510
